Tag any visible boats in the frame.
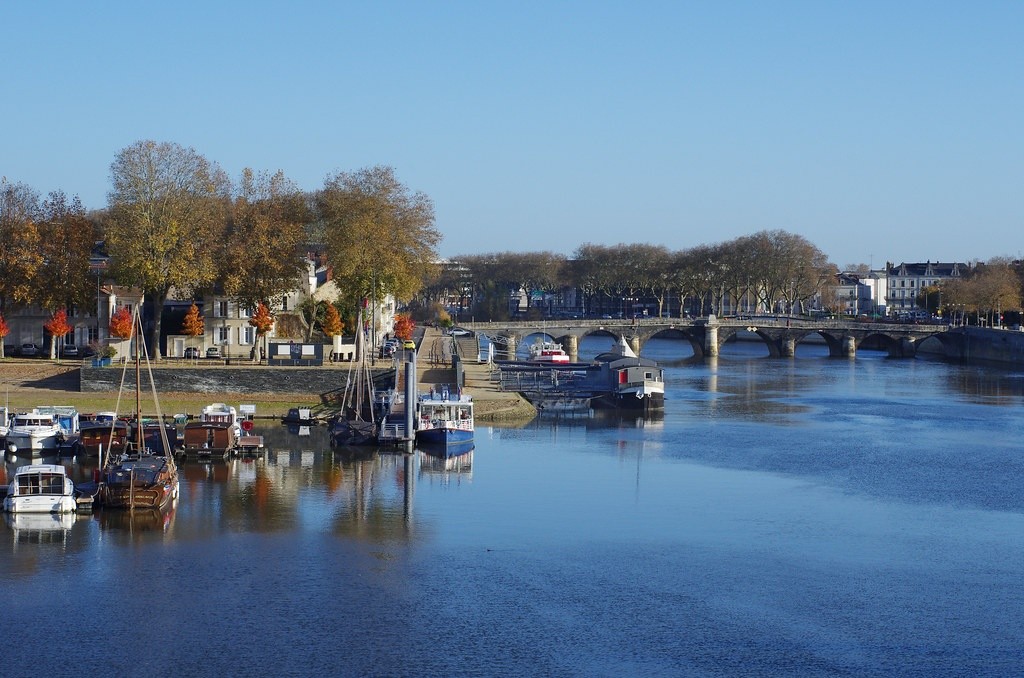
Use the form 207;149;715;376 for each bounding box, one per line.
120;414;178;456
0;406;15;438
182;402;243;462
526;321;569;365
2;463;76;513
588;353;665;402
9;406;80;451
78;411;132;456
415;382;475;459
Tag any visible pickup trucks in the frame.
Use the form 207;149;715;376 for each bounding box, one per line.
183;347;199;358
205;347;222;357
249;347;265;359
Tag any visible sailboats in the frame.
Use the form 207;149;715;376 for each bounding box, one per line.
326;309;379;448
95;304;180;511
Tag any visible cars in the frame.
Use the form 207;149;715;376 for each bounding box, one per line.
447;329;472;338
402;340;416;350
378;337;399;358
545;309;654;318
63;345;79;355
22;343;40;355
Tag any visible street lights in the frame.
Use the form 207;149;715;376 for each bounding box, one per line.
747;275;752;314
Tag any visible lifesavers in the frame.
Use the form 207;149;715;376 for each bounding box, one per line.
242;421;253;431
648;394;651;397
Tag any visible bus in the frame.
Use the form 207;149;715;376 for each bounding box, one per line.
808;309;833;317
830;313;868;321
